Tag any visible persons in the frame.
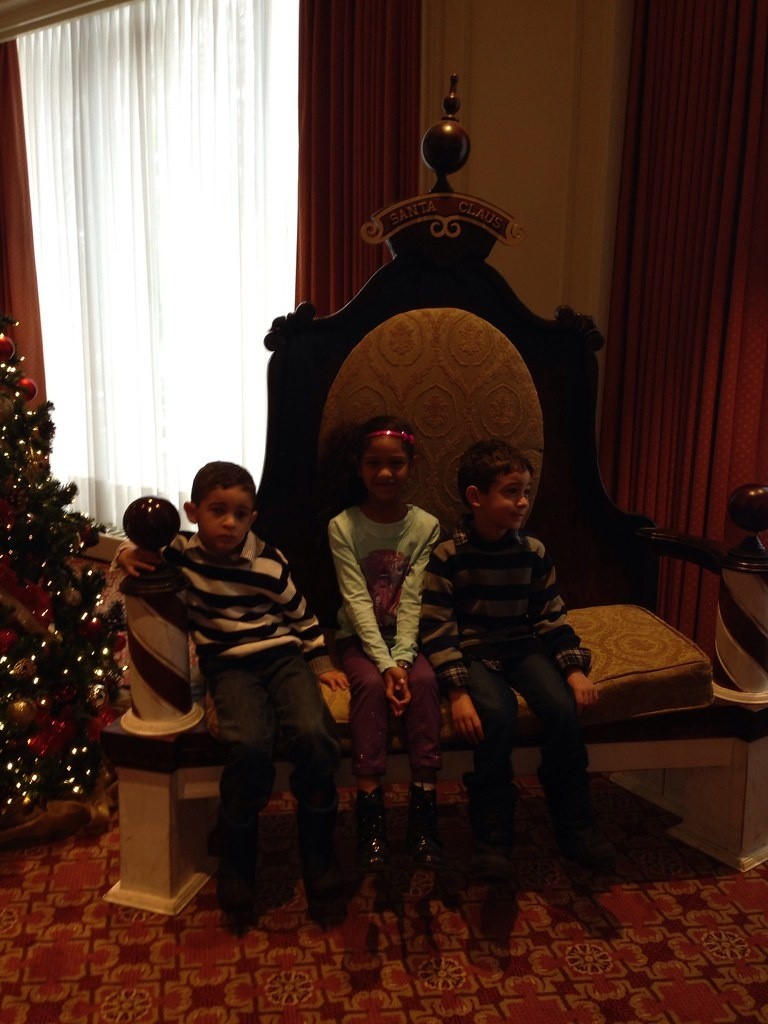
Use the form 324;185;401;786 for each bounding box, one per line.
420;438;614;867
116;463;351;910
326;417;442;872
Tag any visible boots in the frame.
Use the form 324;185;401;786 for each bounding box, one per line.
357;782;389;866
214;805;261;912
466;771;518;853
401;781;443;869
298;790;348;923
536;766;602;869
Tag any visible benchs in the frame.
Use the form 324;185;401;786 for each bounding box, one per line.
104;73;768;915
